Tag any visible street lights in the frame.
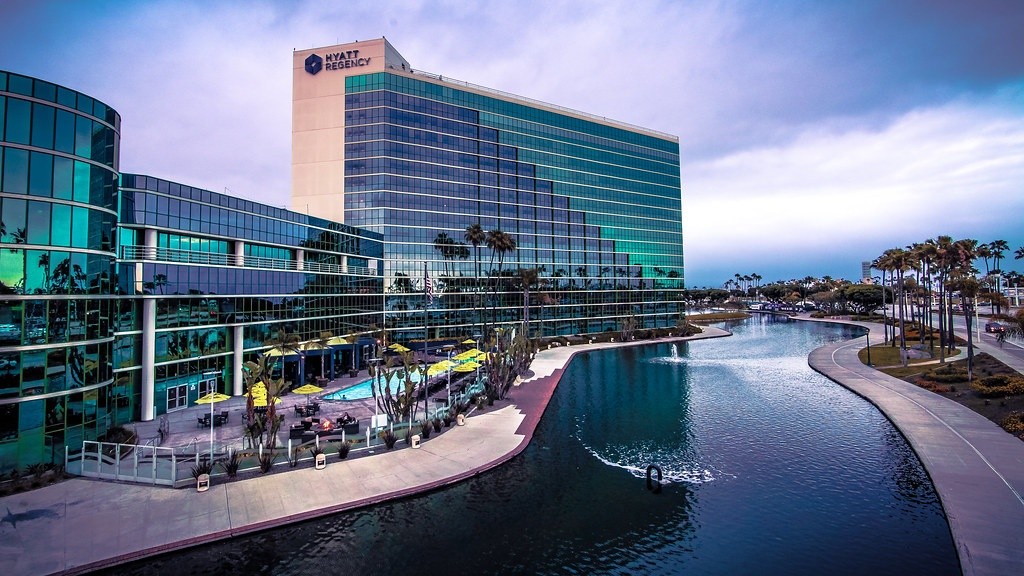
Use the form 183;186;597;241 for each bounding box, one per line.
369;358;383;438
443;345;456;409
473;335;482;383
202;370;222;463
864;328;870;365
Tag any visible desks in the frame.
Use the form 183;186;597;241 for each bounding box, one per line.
208;415;221;425
309;425;334;436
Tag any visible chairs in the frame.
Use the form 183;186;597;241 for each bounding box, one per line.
241;403;359;444
197;411;228;430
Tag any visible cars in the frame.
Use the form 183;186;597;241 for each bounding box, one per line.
814;302;889;311
927;303;944;312
980;302;990;306
724;300;798;312
953;303;978;312
914;304;929;316
985;322;1004;333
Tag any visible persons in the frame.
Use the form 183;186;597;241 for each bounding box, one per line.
996;331;1005;343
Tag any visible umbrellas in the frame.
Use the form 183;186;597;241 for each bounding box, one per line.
297;343;333;350
194;392;231;404
263;347;303;356
243;380;282;406
292;384;324;405
426;349;496;383
388;343;410;352
325;338;358;345
462;339;476;343
